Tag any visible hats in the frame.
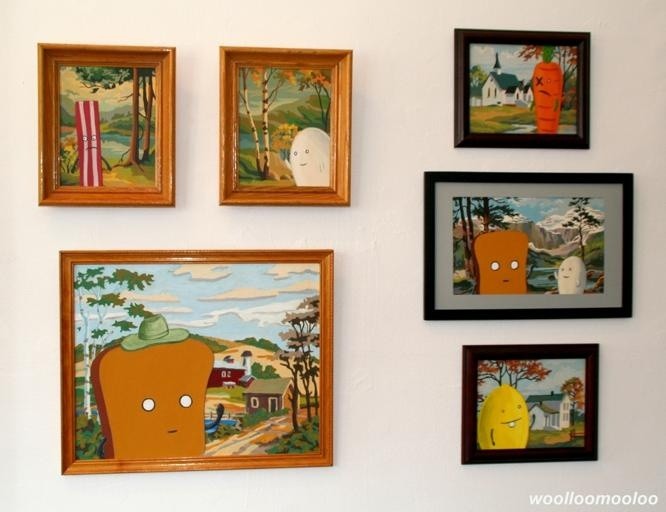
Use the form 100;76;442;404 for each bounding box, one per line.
120;313;189;352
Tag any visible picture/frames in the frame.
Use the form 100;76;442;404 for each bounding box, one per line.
424;171;633;320
454;28;590;150
36;41;176;208
219;45;354;207
58;249;334;476
462;344;599;464
